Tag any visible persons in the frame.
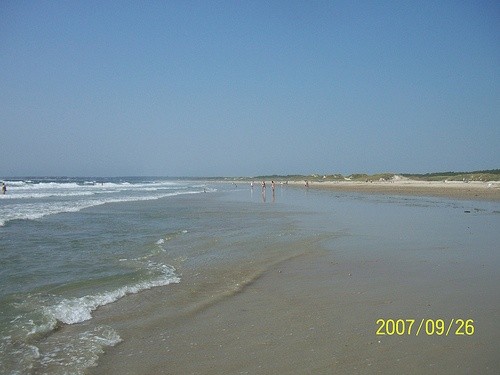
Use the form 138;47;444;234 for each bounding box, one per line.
247;179;311;190
